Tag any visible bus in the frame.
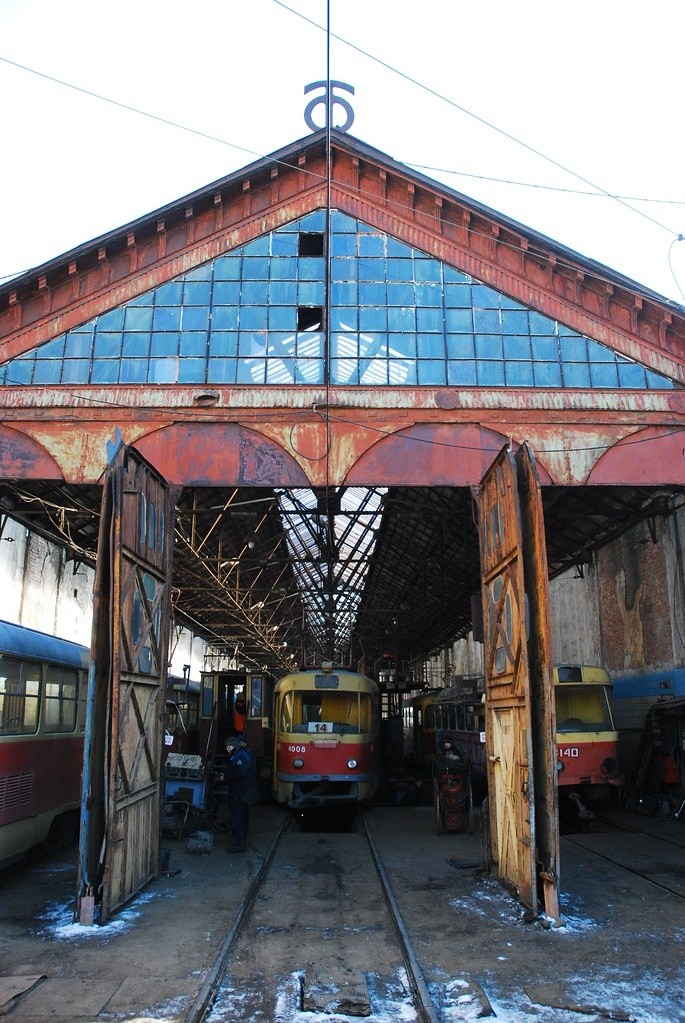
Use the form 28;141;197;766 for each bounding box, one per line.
400;666;624;820
271;662;382;824
197;670;279;777
1;618;205;874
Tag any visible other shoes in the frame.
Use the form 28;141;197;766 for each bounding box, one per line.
227;843;246;852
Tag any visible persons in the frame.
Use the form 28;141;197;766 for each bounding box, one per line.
218;736;259;852
442;736;461;775
400;778;424;806
234;692;246;733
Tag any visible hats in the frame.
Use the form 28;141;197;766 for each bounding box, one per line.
224;736;238;747
